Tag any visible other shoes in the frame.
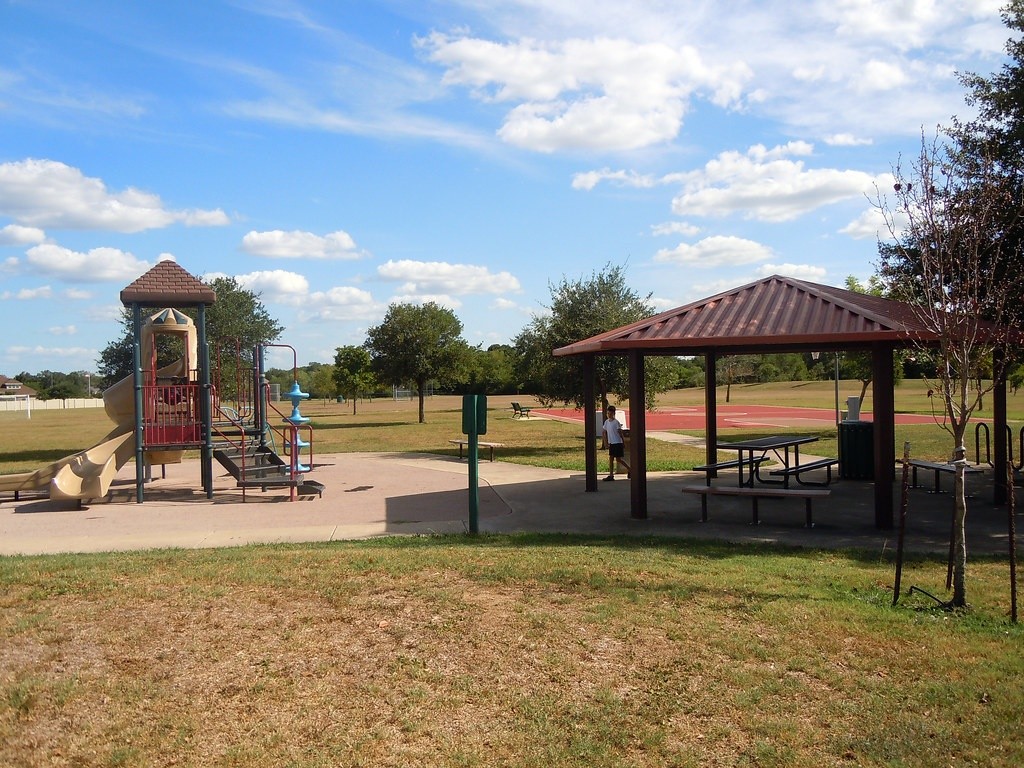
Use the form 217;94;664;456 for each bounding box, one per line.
627;471;631;479
603;476;615;481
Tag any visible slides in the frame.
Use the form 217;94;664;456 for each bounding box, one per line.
0;356;188;502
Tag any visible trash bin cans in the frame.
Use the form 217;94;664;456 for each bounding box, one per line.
837;420;875;479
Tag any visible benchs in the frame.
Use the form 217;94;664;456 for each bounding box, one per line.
681;485;832;530
448;440;506;462
692;434;839;488
511;402;531;419
895;458;985;493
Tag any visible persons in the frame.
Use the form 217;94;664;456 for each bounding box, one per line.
602;406;631;481
